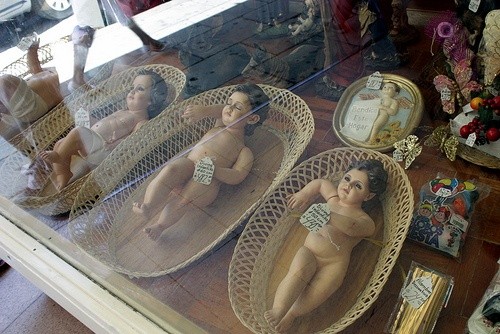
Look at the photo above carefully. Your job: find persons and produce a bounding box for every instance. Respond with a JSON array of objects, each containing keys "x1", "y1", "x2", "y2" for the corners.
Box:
[
  {"x1": 132, "y1": 83, "x2": 270, "y2": 241},
  {"x1": 288, "y1": 6, "x2": 316, "y2": 35},
  {"x1": 266, "y1": 157, "x2": 389, "y2": 331},
  {"x1": 40, "y1": 70, "x2": 167, "y2": 192},
  {"x1": 360, "y1": 80, "x2": 414, "y2": 144}
]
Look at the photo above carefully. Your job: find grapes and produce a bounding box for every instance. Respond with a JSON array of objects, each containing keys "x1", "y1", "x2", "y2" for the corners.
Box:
[{"x1": 475, "y1": 137, "x2": 489, "y2": 146}]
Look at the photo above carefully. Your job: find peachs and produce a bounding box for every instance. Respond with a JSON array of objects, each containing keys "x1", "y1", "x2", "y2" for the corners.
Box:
[{"x1": 470, "y1": 97, "x2": 485, "y2": 110}]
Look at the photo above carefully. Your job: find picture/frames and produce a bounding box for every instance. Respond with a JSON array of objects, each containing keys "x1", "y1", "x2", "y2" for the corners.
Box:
[{"x1": 332, "y1": 74, "x2": 425, "y2": 154}]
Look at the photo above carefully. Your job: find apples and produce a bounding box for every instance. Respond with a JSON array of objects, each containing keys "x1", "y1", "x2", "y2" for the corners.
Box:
[{"x1": 484, "y1": 96, "x2": 500, "y2": 113}]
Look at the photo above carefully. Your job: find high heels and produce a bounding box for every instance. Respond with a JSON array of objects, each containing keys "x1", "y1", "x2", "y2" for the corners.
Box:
[
  {"x1": 146, "y1": 41, "x2": 172, "y2": 57},
  {"x1": 65, "y1": 80, "x2": 96, "y2": 94}
]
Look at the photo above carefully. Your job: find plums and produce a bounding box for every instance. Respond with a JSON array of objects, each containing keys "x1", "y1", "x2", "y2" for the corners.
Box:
[{"x1": 460, "y1": 117, "x2": 499, "y2": 141}]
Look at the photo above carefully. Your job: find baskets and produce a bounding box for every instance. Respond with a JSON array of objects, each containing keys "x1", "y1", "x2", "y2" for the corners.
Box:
[
  {"x1": 66, "y1": 84, "x2": 315, "y2": 277},
  {"x1": 1, "y1": 25, "x2": 117, "y2": 148},
  {"x1": 229, "y1": 147, "x2": 415, "y2": 334},
  {"x1": 0, "y1": 64, "x2": 187, "y2": 218}
]
